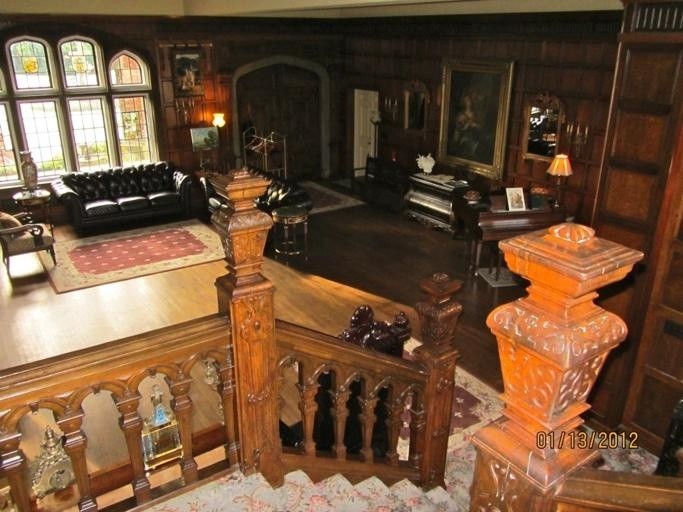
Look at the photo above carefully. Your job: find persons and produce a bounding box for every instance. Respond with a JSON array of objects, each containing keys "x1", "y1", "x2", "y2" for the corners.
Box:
[
  {"x1": 453, "y1": 94, "x2": 490, "y2": 164},
  {"x1": 510, "y1": 192, "x2": 523, "y2": 208}
]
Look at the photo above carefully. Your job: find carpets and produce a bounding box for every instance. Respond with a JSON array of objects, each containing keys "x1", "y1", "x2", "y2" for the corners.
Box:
[
  {"x1": 478, "y1": 267, "x2": 519, "y2": 287},
  {"x1": 365, "y1": 320, "x2": 505, "y2": 462},
  {"x1": 37, "y1": 217, "x2": 226, "y2": 294},
  {"x1": 286, "y1": 180, "x2": 368, "y2": 216}
]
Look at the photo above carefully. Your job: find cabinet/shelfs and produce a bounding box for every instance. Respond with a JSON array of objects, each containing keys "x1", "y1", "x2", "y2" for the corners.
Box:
[{"x1": 244, "y1": 126, "x2": 287, "y2": 179}]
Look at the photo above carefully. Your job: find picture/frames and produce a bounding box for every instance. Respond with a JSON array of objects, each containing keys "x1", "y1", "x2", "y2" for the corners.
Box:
[
  {"x1": 170, "y1": 46, "x2": 204, "y2": 97},
  {"x1": 505, "y1": 187, "x2": 526, "y2": 211},
  {"x1": 435, "y1": 58, "x2": 516, "y2": 182}
]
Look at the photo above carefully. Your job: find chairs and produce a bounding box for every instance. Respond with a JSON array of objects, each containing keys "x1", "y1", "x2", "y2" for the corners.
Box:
[{"x1": 0, "y1": 211, "x2": 56, "y2": 281}]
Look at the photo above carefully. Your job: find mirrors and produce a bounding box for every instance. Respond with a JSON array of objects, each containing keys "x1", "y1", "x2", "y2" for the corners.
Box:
[
  {"x1": 521, "y1": 93, "x2": 562, "y2": 163},
  {"x1": 402, "y1": 76, "x2": 431, "y2": 143}
]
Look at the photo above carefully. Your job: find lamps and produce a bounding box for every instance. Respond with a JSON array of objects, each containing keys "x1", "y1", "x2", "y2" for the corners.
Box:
[{"x1": 546, "y1": 153, "x2": 574, "y2": 209}]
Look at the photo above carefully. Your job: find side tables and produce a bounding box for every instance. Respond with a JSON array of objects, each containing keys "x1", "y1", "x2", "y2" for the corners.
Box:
[
  {"x1": 271, "y1": 204, "x2": 312, "y2": 265},
  {"x1": 12, "y1": 188, "x2": 51, "y2": 213}
]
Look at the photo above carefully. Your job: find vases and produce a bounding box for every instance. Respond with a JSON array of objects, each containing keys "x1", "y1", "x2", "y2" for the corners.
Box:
[{"x1": 20, "y1": 151, "x2": 37, "y2": 190}]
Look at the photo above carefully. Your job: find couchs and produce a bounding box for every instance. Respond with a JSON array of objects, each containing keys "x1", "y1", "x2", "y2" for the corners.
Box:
[
  {"x1": 49, "y1": 161, "x2": 204, "y2": 237},
  {"x1": 206, "y1": 165, "x2": 313, "y2": 224}
]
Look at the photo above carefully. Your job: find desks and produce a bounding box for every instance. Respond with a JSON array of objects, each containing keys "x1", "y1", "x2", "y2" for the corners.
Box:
[{"x1": 403, "y1": 172, "x2": 482, "y2": 240}]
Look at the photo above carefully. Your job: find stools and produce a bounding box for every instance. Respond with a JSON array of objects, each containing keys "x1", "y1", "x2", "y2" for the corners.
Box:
[{"x1": 489, "y1": 247, "x2": 505, "y2": 282}]
